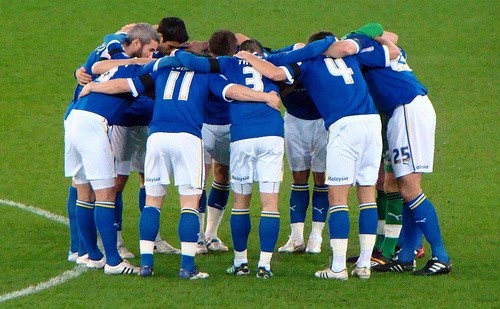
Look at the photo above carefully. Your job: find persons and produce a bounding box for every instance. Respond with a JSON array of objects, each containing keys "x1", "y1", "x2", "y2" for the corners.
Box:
[{"x1": 63, "y1": 16, "x2": 453, "y2": 280}]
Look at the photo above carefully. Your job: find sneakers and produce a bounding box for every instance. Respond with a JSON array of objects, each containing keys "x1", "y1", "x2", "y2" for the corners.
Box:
[
  {"x1": 205, "y1": 237, "x2": 229, "y2": 251},
  {"x1": 372, "y1": 260, "x2": 416, "y2": 272},
  {"x1": 196, "y1": 240, "x2": 208, "y2": 253},
  {"x1": 86, "y1": 256, "x2": 105, "y2": 269},
  {"x1": 348, "y1": 250, "x2": 382, "y2": 263},
  {"x1": 256, "y1": 267, "x2": 273, "y2": 278},
  {"x1": 154, "y1": 240, "x2": 181, "y2": 255},
  {"x1": 351, "y1": 268, "x2": 371, "y2": 279},
  {"x1": 352, "y1": 254, "x2": 392, "y2": 268},
  {"x1": 118, "y1": 246, "x2": 135, "y2": 259},
  {"x1": 69, "y1": 251, "x2": 78, "y2": 262},
  {"x1": 139, "y1": 266, "x2": 154, "y2": 276},
  {"x1": 416, "y1": 247, "x2": 425, "y2": 259},
  {"x1": 179, "y1": 268, "x2": 210, "y2": 279},
  {"x1": 414, "y1": 256, "x2": 453, "y2": 276},
  {"x1": 306, "y1": 235, "x2": 322, "y2": 253},
  {"x1": 227, "y1": 263, "x2": 250, "y2": 275},
  {"x1": 277, "y1": 236, "x2": 306, "y2": 253},
  {"x1": 76, "y1": 254, "x2": 88, "y2": 265},
  {"x1": 315, "y1": 267, "x2": 348, "y2": 280},
  {"x1": 104, "y1": 260, "x2": 140, "y2": 274}
]
